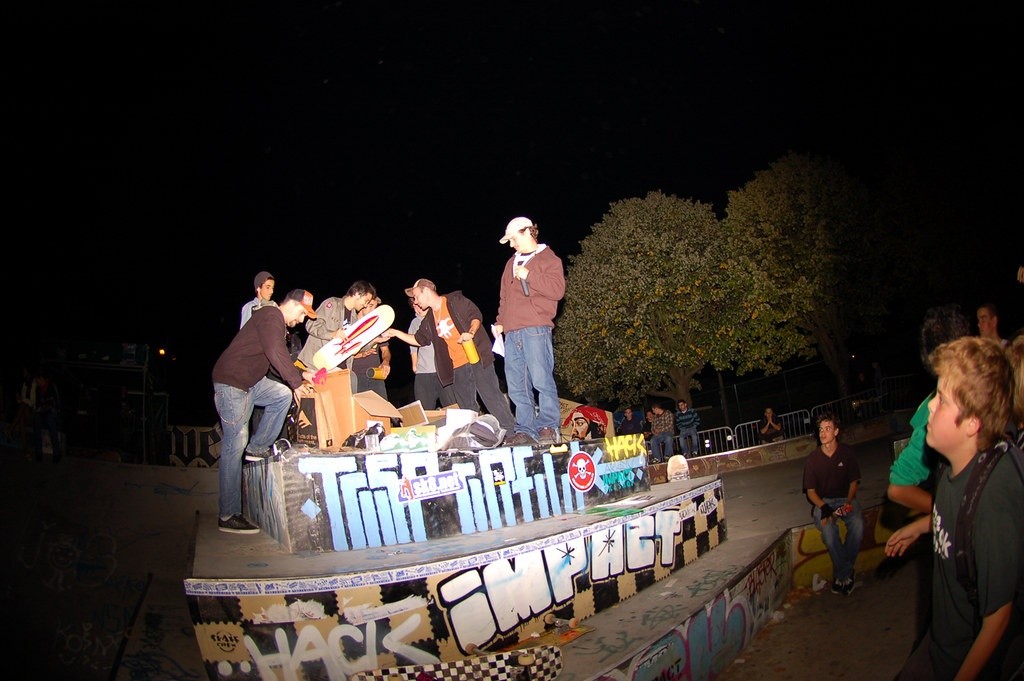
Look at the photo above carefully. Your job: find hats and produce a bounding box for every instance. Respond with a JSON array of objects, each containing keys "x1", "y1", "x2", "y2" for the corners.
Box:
[
  {"x1": 405, "y1": 279, "x2": 436, "y2": 298},
  {"x1": 254, "y1": 271, "x2": 274, "y2": 290},
  {"x1": 287, "y1": 288, "x2": 318, "y2": 319},
  {"x1": 499, "y1": 217, "x2": 532, "y2": 244}
]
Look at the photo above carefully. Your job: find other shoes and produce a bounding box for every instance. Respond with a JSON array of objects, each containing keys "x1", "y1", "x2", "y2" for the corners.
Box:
[
  {"x1": 684, "y1": 454, "x2": 688, "y2": 459},
  {"x1": 665, "y1": 457, "x2": 669, "y2": 463},
  {"x1": 653, "y1": 458, "x2": 658, "y2": 463},
  {"x1": 692, "y1": 453, "x2": 697, "y2": 457}
]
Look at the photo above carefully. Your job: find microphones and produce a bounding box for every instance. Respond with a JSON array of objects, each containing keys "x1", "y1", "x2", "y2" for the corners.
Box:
[{"x1": 520, "y1": 278, "x2": 530, "y2": 297}]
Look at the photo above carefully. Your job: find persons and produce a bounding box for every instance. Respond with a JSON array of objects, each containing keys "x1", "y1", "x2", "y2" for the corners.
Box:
[
  {"x1": 617, "y1": 407, "x2": 641, "y2": 435},
  {"x1": 803, "y1": 412, "x2": 864, "y2": 596},
  {"x1": 642, "y1": 410, "x2": 654, "y2": 442},
  {"x1": 212, "y1": 289, "x2": 318, "y2": 535},
  {"x1": 674, "y1": 399, "x2": 701, "y2": 459},
  {"x1": 651, "y1": 404, "x2": 675, "y2": 463},
  {"x1": 491, "y1": 217, "x2": 566, "y2": 447},
  {"x1": 240, "y1": 271, "x2": 289, "y2": 452},
  {"x1": 758, "y1": 407, "x2": 785, "y2": 445},
  {"x1": 408, "y1": 298, "x2": 457, "y2": 410},
  {"x1": 380, "y1": 279, "x2": 517, "y2": 439},
  {"x1": 883, "y1": 267, "x2": 1024, "y2": 681},
  {"x1": 1, "y1": 373, "x2": 59, "y2": 464},
  {"x1": 351, "y1": 297, "x2": 391, "y2": 400},
  {"x1": 297, "y1": 279, "x2": 377, "y2": 372}
]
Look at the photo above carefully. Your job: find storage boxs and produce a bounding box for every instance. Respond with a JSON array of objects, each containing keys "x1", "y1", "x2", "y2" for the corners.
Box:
[{"x1": 294, "y1": 369, "x2": 459, "y2": 448}]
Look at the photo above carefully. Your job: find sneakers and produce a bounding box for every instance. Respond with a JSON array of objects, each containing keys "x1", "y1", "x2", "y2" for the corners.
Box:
[
  {"x1": 246, "y1": 451, "x2": 277, "y2": 463},
  {"x1": 830, "y1": 578, "x2": 843, "y2": 594},
  {"x1": 218, "y1": 513, "x2": 260, "y2": 534},
  {"x1": 538, "y1": 427, "x2": 556, "y2": 444},
  {"x1": 844, "y1": 569, "x2": 856, "y2": 596},
  {"x1": 502, "y1": 432, "x2": 539, "y2": 446}
]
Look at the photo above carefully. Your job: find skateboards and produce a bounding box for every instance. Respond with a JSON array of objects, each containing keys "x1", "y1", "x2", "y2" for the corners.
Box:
[
  {"x1": 313, "y1": 305, "x2": 395, "y2": 371},
  {"x1": 666, "y1": 454, "x2": 690, "y2": 482}
]
